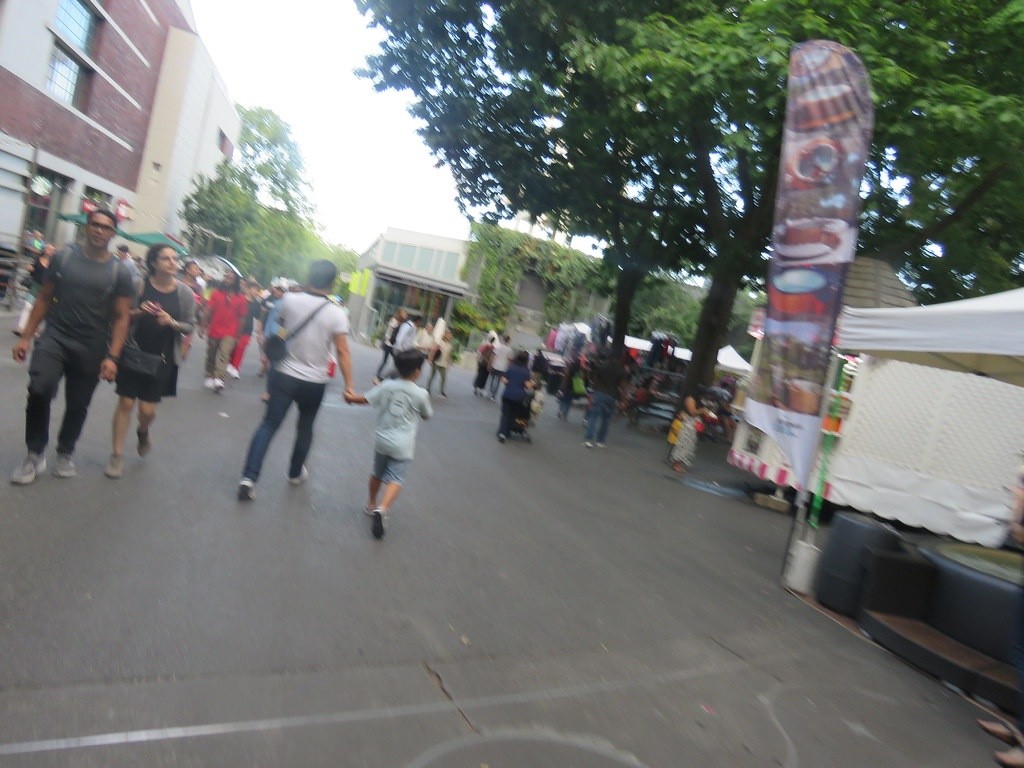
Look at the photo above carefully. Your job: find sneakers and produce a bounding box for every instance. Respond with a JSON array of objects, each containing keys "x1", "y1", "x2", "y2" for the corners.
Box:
[{"x1": 10, "y1": 453, "x2": 46, "y2": 485}]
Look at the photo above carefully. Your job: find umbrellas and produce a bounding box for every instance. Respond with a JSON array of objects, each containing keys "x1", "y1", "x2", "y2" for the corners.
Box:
[{"x1": 56, "y1": 213, "x2": 190, "y2": 256}]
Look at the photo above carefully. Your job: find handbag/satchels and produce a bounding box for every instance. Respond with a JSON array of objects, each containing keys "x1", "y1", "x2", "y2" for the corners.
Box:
[{"x1": 263, "y1": 336, "x2": 288, "y2": 363}]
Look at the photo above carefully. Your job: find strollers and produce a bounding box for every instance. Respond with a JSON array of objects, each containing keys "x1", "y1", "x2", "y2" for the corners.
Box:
[{"x1": 511, "y1": 377, "x2": 543, "y2": 442}]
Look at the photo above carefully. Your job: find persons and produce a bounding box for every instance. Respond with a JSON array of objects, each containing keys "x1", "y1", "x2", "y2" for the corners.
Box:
[
  {"x1": 531, "y1": 349, "x2": 546, "y2": 390},
  {"x1": 496, "y1": 351, "x2": 534, "y2": 441},
  {"x1": 665, "y1": 384, "x2": 710, "y2": 472},
  {"x1": 374, "y1": 309, "x2": 452, "y2": 398},
  {"x1": 11, "y1": 209, "x2": 133, "y2": 484},
  {"x1": 344, "y1": 349, "x2": 433, "y2": 539},
  {"x1": 979, "y1": 474, "x2": 1024, "y2": 768},
  {"x1": 707, "y1": 373, "x2": 738, "y2": 442},
  {"x1": 174, "y1": 262, "x2": 302, "y2": 401},
  {"x1": 473, "y1": 335, "x2": 512, "y2": 402},
  {"x1": 556, "y1": 351, "x2": 670, "y2": 448},
  {"x1": 238, "y1": 260, "x2": 355, "y2": 500},
  {"x1": 106, "y1": 243, "x2": 195, "y2": 479},
  {"x1": 116, "y1": 245, "x2": 148, "y2": 283},
  {"x1": 12, "y1": 225, "x2": 55, "y2": 338}
]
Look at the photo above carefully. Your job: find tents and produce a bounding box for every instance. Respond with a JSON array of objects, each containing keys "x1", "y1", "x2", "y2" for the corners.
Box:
[
  {"x1": 805, "y1": 289, "x2": 1024, "y2": 543},
  {"x1": 716, "y1": 345, "x2": 753, "y2": 378}
]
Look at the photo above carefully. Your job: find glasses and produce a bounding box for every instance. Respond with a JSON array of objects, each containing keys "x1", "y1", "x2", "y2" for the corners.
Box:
[{"x1": 88, "y1": 221, "x2": 117, "y2": 236}]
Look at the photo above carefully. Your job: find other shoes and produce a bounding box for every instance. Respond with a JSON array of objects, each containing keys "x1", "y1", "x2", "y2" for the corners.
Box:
[
  {"x1": 237, "y1": 480, "x2": 255, "y2": 501},
  {"x1": 214, "y1": 378, "x2": 224, "y2": 390},
  {"x1": 137, "y1": 428, "x2": 150, "y2": 456},
  {"x1": 364, "y1": 505, "x2": 377, "y2": 517},
  {"x1": 286, "y1": 466, "x2": 308, "y2": 484},
  {"x1": 105, "y1": 454, "x2": 122, "y2": 477},
  {"x1": 204, "y1": 379, "x2": 214, "y2": 390},
  {"x1": 371, "y1": 510, "x2": 387, "y2": 540},
  {"x1": 226, "y1": 366, "x2": 240, "y2": 378}
]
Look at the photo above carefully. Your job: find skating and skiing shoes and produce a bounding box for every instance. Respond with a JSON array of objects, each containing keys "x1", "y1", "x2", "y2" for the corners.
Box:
[{"x1": 55, "y1": 453, "x2": 76, "y2": 477}]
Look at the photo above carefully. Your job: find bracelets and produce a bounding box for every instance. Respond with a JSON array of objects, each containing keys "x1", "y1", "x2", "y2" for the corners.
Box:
[{"x1": 105, "y1": 354, "x2": 120, "y2": 364}]
